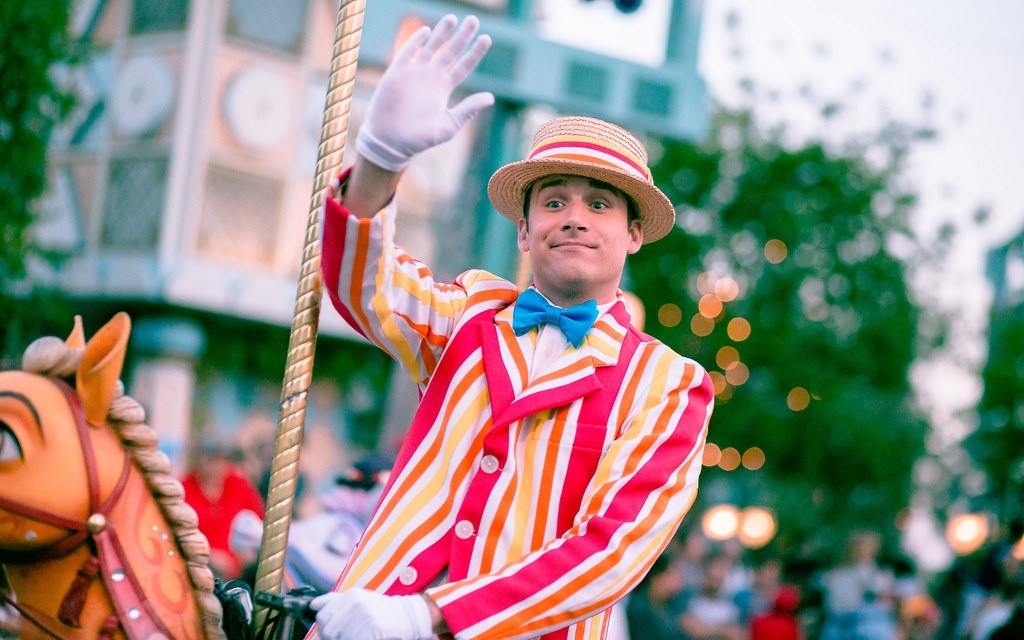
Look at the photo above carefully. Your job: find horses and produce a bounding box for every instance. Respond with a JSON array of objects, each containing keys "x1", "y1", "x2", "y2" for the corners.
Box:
[{"x1": 0, "y1": 312, "x2": 226, "y2": 640}]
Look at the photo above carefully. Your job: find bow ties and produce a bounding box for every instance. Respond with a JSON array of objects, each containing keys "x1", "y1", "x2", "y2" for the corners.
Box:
[{"x1": 512, "y1": 287, "x2": 604, "y2": 347}]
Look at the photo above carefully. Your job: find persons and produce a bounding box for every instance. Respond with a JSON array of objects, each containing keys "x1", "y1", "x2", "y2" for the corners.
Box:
[
  {"x1": 629, "y1": 515, "x2": 1024, "y2": 640},
  {"x1": 304, "y1": 12, "x2": 716, "y2": 640},
  {"x1": 181, "y1": 416, "x2": 307, "y2": 582}
]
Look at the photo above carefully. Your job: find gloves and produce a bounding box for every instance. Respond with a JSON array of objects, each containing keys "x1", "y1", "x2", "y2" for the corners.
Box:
[
  {"x1": 355, "y1": 12, "x2": 499, "y2": 174},
  {"x1": 309, "y1": 587, "x2": 435, "y2": 640}
]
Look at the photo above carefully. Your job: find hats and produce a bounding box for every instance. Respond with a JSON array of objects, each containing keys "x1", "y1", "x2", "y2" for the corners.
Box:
[{"x1": 485, "y1": 114, "x2": 677, "y2": 244}]
